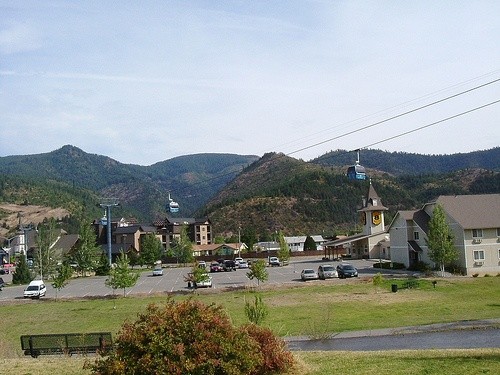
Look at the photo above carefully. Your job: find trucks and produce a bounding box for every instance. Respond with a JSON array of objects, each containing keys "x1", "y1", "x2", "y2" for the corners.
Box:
[{"x1": 269, "y1": 257, "x2": 280, "y2": 267}]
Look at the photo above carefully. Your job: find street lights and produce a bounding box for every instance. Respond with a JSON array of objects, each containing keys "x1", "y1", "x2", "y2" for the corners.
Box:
[{"x1": 0, "y1": 236, "x2": 21, "y2": 264}]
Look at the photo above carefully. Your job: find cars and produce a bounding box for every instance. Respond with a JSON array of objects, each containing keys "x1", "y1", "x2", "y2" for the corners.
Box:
[
  {"x1": 152, "y1": 267, "x2": 163, "y2": 276},
  {"x1": 198, "y1": 261, "x2": 207, "y2": 268},
  {"x1": 301, "y1": 268, "x2": 318, "y2": 281},
  {"x1": 70, "y1": 262, "x2": 79, "y2": 270},
  {"x1": 193, "y1": 273, "x2": 213, "y2": 288},
  {"x1": 223, "y1": 257, "x2": 249, "y2": 272},
  {"x1": 27, "y1": 260, "x2": 34, "y2": 268}
]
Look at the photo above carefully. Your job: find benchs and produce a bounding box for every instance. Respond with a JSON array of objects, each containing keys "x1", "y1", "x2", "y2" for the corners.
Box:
[{"x1": 20, "y1": 331, "x2": 118, "y2": 359}]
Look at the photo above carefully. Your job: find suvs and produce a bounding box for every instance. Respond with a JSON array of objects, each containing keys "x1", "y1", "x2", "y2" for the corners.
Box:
[
  {"x1": 23, "y1": 280, "x2": 47, "y2": 299},
  {"x1": 318, "y1": 263, "x2": 339, "y2": 280},
  {"x1": 336, "y1": 262, "x2": 358, "y2": 279},
  {"x1": 209, "y1": 262, "x2": 222, "y2": 273}
]
